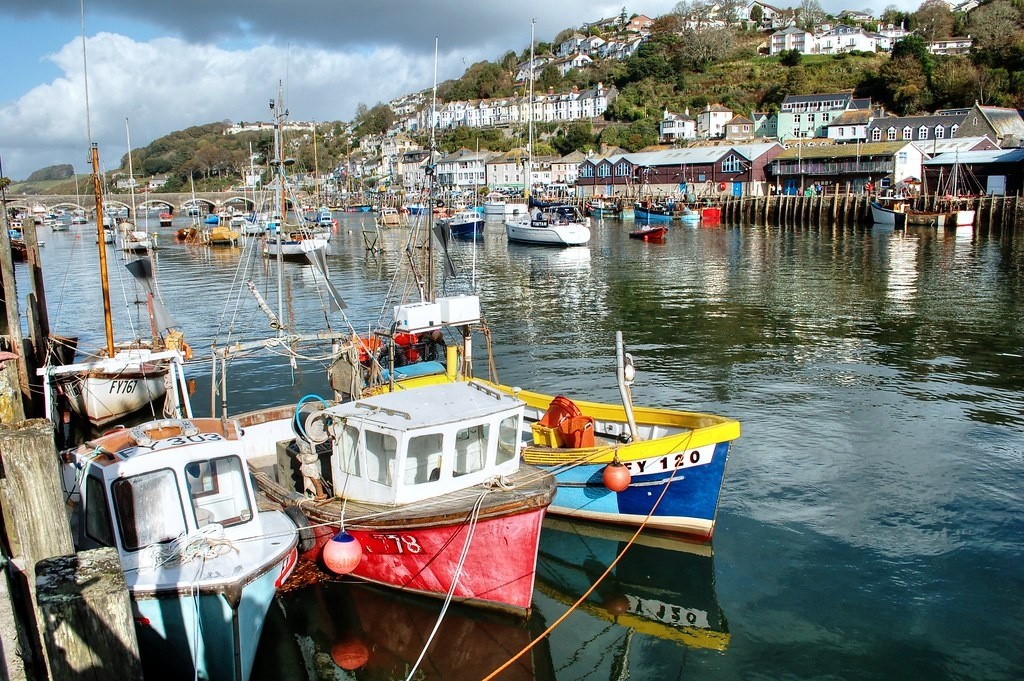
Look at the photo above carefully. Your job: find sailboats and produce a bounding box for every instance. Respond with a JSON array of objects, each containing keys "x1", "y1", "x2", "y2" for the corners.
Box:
[
  {"x1": 114, "y1": 113, "x2": 159, "y2": 251},
  {"x1": 56, "y1": 1, "x2": 185, "y2": 428},
  {"x1": 260, "y1": 79, "x2": 330, "y2": 259}
]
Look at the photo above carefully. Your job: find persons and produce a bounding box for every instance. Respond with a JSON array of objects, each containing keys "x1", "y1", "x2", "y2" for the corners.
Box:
[
  {"x1": 810, "y1": 182, "x2": 823, "y2": 196},
  {"x1": 0, "y1": 351, "x2": 20, "y2": 370},
  {"x1": 863, "y1": 182, "x2": 873, "y2": 196}
]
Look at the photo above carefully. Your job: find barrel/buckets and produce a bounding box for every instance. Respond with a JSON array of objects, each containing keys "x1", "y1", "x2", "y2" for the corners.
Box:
[
  {"x1": 537, "y1": 394, "x2": 582, "y2": 427},
  {"x1": 558, "y1": 416, "x2": 595, "y2": 448}
]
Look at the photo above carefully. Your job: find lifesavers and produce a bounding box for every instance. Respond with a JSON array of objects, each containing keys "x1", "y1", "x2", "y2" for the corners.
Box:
[
  {"x1": 131, "y1": 417, "x2": 198, "y2": 448},
  {"x1": 284, "y1": 505, "x2": 317, "y2": 551}
]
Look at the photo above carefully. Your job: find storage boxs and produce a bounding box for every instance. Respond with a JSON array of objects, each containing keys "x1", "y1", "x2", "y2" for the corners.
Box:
[
  {"x1": 530, "y1": 422, "x2": 564, "y2": 449},
  {"x1": 436, "y1": 296, "x2": 480, "y2": 326},
  {"x1": 394, "y1": 301, "x2": 441, "y2": 335}
]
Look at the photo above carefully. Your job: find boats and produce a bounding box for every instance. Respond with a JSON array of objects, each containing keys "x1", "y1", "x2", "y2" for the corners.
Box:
[
  {"x1": 290, "y1": 574, "x2": 557, "y2": 681},
  {"x1": 503, "y1": 202, "x2": 590, "y2": 246},
  {"x1": 318, "y1": 37, "x2": 743, "y2": 537},
  {"x1": 435, "y1": 207, "x2": 486, "y2": 241},
  {"x1": 159, "y1": 211, "x2": 174, "y2": 227},
  {"x1": 16, "y1": 19, "x2": 722, "y2": 235},
  {"x1": 210, "y1": 141, "x2": 559, "y2": 617},
  {"x1": 869, "y1": 148, "x2": 985, "y2": 227},
  {"x1": 374, "y1": 207, "x2": 399, "y2": 225},
  {"x1": 51, "y1": 343, "x2": 299, "y2": 681},
  {"x1": 533, "y1": 518, "x2": 733, "y2": 681},
  {"x1": 629, "y1": 225, "x2": 668, "y2": 239}
]
